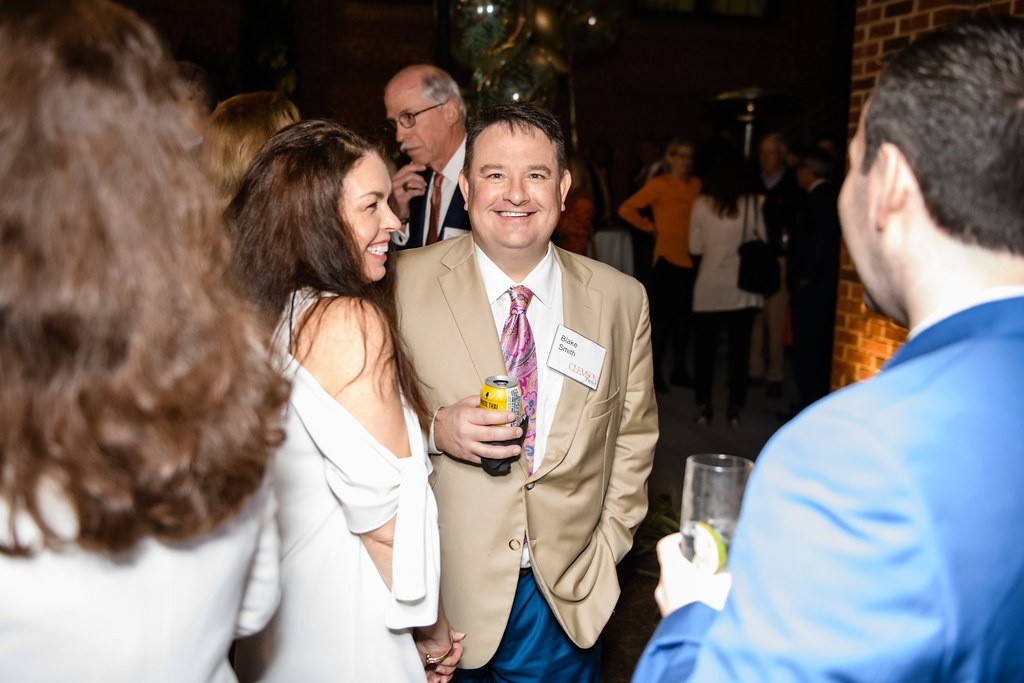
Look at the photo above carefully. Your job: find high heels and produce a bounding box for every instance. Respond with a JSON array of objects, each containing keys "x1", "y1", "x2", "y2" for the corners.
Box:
[
  {"x1": 725, "y1": 406, "x2": 741, "y2": 424},
  {"x1": 693, "y1": 405, "x2": 715, "y2": 426}
]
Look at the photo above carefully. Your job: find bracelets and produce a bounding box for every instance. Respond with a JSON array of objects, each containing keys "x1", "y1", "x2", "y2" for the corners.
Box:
[
  {"x1": 398, "y1": 214, "x2": 411, "y2": 226},
  {"x1": 425, "y1": 627, "x2": 455, "y2": 666}
]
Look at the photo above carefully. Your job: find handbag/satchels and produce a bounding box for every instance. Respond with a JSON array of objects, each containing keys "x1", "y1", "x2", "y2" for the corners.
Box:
[{"x1": 737, "y1": 193, "x2": 782, "y2": 299}]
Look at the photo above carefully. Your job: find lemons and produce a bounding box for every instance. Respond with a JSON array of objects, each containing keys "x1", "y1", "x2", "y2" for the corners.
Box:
[{"x1": 694, "y1": 521, "x2": 725, "y2": 574}]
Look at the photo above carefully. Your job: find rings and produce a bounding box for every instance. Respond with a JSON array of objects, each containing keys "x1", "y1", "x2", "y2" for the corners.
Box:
[{"x1": 403, "y1": 180, "x2": 409, "y2": 192}]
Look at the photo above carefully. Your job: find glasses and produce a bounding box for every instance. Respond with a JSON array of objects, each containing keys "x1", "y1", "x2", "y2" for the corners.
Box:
[{"x1": 384, "y1": 103, "x2": 444, "y2": 133}]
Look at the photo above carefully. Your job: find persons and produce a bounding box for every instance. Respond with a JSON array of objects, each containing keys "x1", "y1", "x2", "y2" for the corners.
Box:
[
  {"x1": 210, "y1": 114, "x2": 466, "y2": 683},
  {"x1": 544, "y1": 128, "x2": 853, "y2": 436},
  {"x1": 629, "y1": 14, "x2": 1024, "y2": 683},
  {"x1": 0, "y1": 2, "x2": 284, "y2": 682},
  {"x1": 198, "y1": 91, "x2": 305, "y2": 243},
  {"x1": 365, "y1": 64, "x2": 490, "y2": 266},
  {"x1": 378, "y1": 99, "x2": 658, "y2": 682}
]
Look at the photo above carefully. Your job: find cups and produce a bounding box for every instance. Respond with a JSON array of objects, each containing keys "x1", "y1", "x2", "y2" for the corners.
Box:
[{"x1": 680, "y1": 454, "x2": 754, "y2": 572}]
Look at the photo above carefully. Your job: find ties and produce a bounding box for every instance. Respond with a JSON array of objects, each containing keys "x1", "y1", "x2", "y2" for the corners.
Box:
[
  {"x1": 425, "y1": 172, "x2": 445, "y2": 246},
  {"x1": 499, "y1": 284, "x2": 538, "y2": 476}
]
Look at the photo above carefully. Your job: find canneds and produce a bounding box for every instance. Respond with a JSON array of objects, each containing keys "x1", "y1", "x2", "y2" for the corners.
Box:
[{"x1": 479, "y1": 375, "x2": 521, "y2": 429}]
